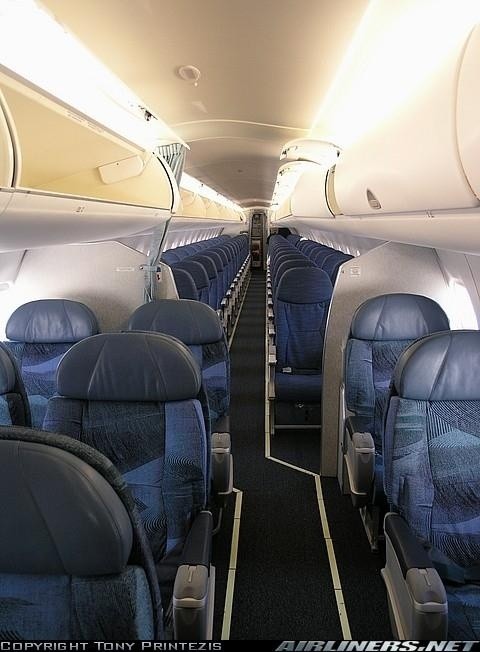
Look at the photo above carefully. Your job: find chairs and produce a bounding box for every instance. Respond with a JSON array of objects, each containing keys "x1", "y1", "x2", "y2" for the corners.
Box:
[{"x1": 0, "y1": 234, "x2": 480, "y2": 652}]
